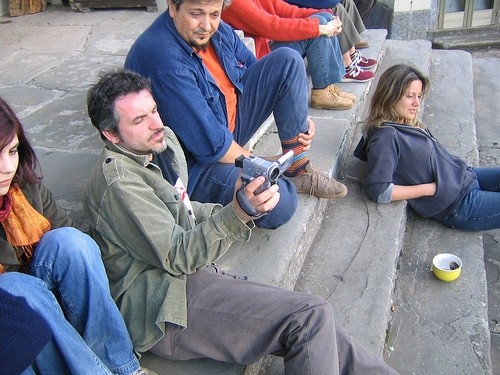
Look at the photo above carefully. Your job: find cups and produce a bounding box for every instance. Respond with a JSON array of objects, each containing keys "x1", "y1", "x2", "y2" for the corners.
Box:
[{"x1": 430, "y1": 253, "x2": 462, "y2": 282}]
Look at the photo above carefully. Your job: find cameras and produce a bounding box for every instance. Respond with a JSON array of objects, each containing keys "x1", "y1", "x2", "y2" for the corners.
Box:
[{"x1": 235, "y1": 149, "x2": 294, "y2": 196}]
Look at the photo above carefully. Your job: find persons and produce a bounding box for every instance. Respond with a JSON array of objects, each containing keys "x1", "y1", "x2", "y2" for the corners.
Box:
[
  {"x1": 221, "y1": 0, "x2": 357, "y2": 111},
  {"x1": 83, "y1": 69, "x2": 401, "y2": 374},
  {"x1": 353, "y1": 65, "x2": 500, "y2": 232},
  {"x1": 0, "y1": 96, "x2": 157, "y2": 375},
  {"x1": 288, "y1": 0, "x2": 377, "y2": 81},
  {"x1": 125, "y1": 0, "x2": 347, "y2": 230}
]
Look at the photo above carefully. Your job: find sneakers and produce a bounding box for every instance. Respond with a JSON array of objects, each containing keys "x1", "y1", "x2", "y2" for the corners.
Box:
[
  {"x1": 355, "y1": 39, "x2": 370, "y2": 49},
  {"x1": 294, "y1": 163, "x2": 348, "y2": 199},
  {"x1": 310, "y1": 85, "x2": 354, "y2": 110},
  {"x1": 331, "y1": 83, "x2": 357, "y2": 102},
  {"x1": 355, "y1": 53, "x2": 378, "y2": 69},
  {"x1": 342, "y1": 63, "x2": 374, "y2": 83}
]
